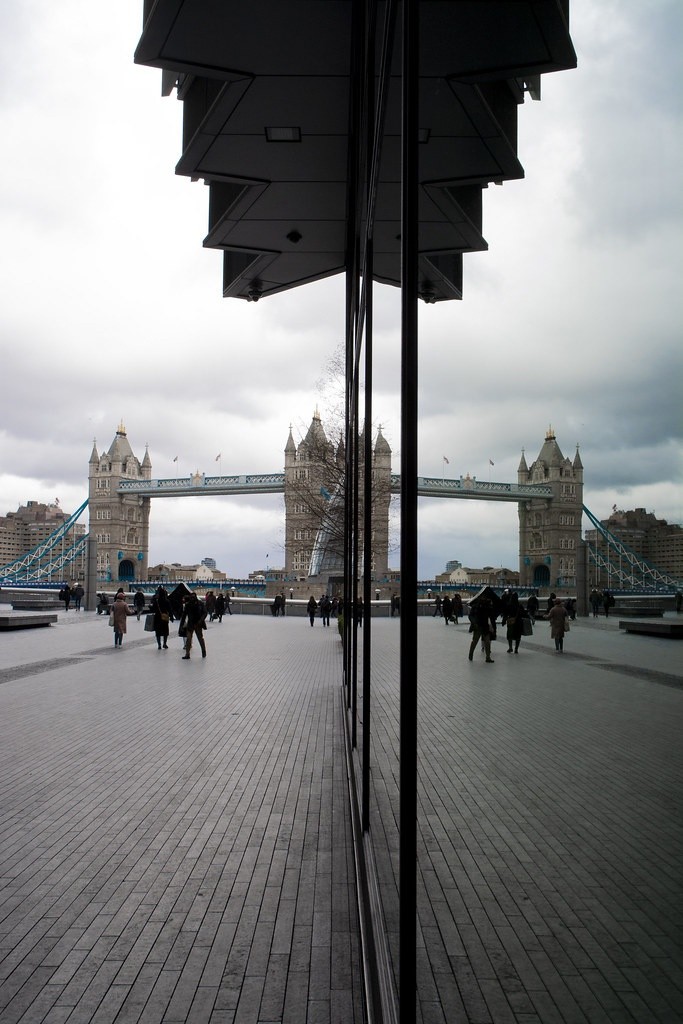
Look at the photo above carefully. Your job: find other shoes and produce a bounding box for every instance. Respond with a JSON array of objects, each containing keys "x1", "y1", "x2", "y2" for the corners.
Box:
[
  {"x1": 202, "y1": 647, "x2": 206, "y2": 657},
  {"x1": 115, "y1": 644, "x2": 117, "y2": 648},
  {"x1": 507, "y1": 648, "x2": 513, "y2": 652},
  {"x1": 469, "y1": 651, "x2": 473, "y2": 661},
  {"x1": 158, "y1": 646, "x2": 161, "y2": 649},
  {"x1": 163, "y1": 645, "x2": 168, "y2": 649},
  {"x1": 515, "y1": 649, "x2": 518, "y2": 653},
  {"x1": 182, "y1": 655, "x2": 190, "y2": 659},
  {"x1": 119, "y1": 646, "x2": 122, "y2": 649},
  {"x1": 485, "y1": 658, "x2": 495, "y2": 662}
]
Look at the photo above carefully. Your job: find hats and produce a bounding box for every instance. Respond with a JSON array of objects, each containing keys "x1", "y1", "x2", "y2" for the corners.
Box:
[{"x1": 117, "y1": 592, "x2": 126, "y2": 600}]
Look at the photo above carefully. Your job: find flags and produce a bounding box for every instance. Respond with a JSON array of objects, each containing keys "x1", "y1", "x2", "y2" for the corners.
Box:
[
  {"x1": 173, "y1": 456, "x2": 178, "y2": 463},
  {"x1": 490, "y1": 460, "x2": 494, "y2": 466},
  {"x1": 54, "y1": 498, "x2": 60, "y2": 506},
  {"x1": 215, "y1": 453, "x2": 221, "y2": 461},
  {"x1": 444, "y1": 455, "x2": 450, "y2": 464}
]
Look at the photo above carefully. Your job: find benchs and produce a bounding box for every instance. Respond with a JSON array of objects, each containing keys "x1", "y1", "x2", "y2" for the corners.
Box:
[
  {"x1": 103, "y1": 603, "x2": 153, "y2": 615},
  {"x1": 524, "y1": 609, "x2": 547, "y2": 620}
]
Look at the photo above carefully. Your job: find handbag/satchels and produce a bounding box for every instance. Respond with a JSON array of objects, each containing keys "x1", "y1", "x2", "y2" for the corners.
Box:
[
  {"x1": 109, "y1": 611, "x2": 115, "y2": 626},
  {"x1": 144, "y1": 615, "x2": 156, "y2": 631},
  {"x1": 564, "y1": 619, "x2": 570, "y2": 632},
  {"x1": 522, "y1": 612, "x2": 533, "y2": 635},
  {"x1": 162, "y1": 614, "x2": 169, "y2": 621}
]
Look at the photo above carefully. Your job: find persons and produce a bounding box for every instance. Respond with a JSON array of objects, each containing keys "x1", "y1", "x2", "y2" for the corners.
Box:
[
  {"x1": 96, "y1": 587, "x2": 135, "y2": 649},
  {"x1": 307, "y1": 595, "x2": 343, "y2": 627},
  {"x1": 589, "y1": 588, "x2": 612, "y2": 618},
  {"x1": 272, "y1": 591, "x2": 285, "y2": 617},
  {"x1": 432, "y1": 594, "x2": 464, "y2": 625},
  {"x1": 357, "y1": 597, "x2": 363, "y2": 627},
  {"x1": 180, "y1": 595, "x2": 192, "y2": 649},
  {"x1": 675, "y1": 589, "x2": 683, "y2": 614},
  {"x1": 205, "y1": 591, "x2": 217, "y2": 622},
  {"x1": 62, "y1": 584, "x2": 84, "y2": 611},
  {"x1": 180, "y1": 592, "x2": 208, "y2": 659},
  {"x1": 173, "y1": 590, "x2": 182, "y2": 620},
  {"x1": 133, "y1": 587, "x2": 146, "y2": 621},
  {"x1": 390, "y1": 592, "x2": 400, "y2": 617},
  {"x1": 215, "y1": 593, "x2": 226, "y2": 623},
  {"x1": 148, "y1": 589, "x2": 174, "y2": 650},
  {"x1": 501, "y1": 588, "x2": 576, "y2": 654},
  {"x1": 468, "y1": 594, "x2": 497, "y2": 663},
  {"x1": 224, "y1": 593, "x2": 233, "y2": 615},
  {"x1": 150, "y1": 585, "x2": 168, "y2": 605}
]
